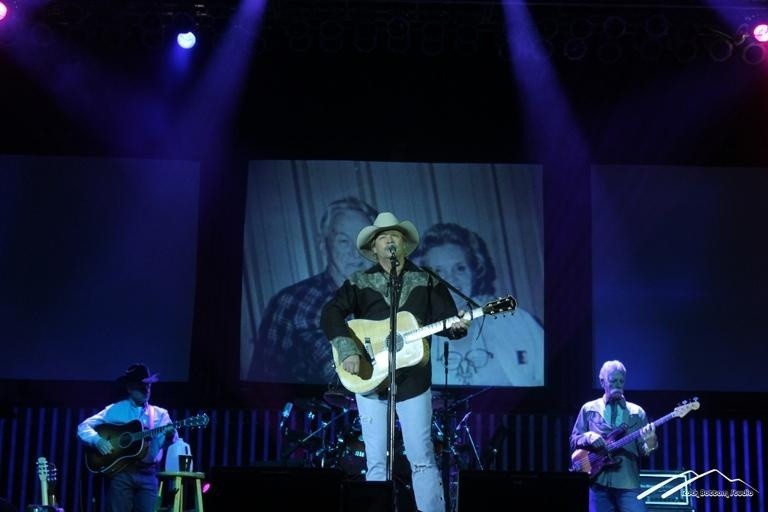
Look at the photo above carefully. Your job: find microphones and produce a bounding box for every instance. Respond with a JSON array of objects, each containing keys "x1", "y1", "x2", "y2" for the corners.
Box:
[{"x1": 385, "y1": 243, "x2": 398, "y2": 258}]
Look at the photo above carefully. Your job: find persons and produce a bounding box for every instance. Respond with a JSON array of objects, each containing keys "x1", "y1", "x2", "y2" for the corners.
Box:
[
  {"x1": 319, "y1": 211, "x2": 473, "y2": 512},
  {"x1": 567, "y1": 359, "x2": 660, "y2": 512},
  {"x1": 77, "y1": 362, "x2": 179, "y2": 511},
  {"x1": 418, "y1": 222, "x2": 545, "y2": 386},
  {"x1": 247, "y1": 194, "x2": 381, "y2": 383}
]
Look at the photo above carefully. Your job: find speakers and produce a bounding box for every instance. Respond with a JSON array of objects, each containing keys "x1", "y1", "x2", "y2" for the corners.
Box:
[
  {"x1": 460, "y1": 471, "x2": 589, "y2": 512},
  {"x1": 340, "y1": 481, "x2": 392, "y2": 512},
  {"x1": 207, "y1": 466, "x2": 345, "y2": 512}
]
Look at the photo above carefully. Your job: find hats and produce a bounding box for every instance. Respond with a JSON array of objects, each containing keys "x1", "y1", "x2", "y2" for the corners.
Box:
[
  {"x1": 117, "y1": 365, "x2": 159, "y2": 384},
  {"x1": 356, "y1": 212, "x2": 420, "y2": 263}
]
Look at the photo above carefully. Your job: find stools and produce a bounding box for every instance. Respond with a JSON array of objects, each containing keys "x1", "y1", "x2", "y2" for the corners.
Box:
[{"x1": 156, "y1": 472, "x2": 205, "y2": 512}]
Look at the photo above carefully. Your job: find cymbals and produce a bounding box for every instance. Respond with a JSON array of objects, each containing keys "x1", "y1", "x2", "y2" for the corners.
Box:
[{"x1": 326, "y1": 394, "x2": 357, "y2": 409}]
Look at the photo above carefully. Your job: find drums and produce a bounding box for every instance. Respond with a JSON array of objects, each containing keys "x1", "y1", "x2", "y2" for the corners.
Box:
[
  {"x1": 336, "y1": 429, "x2": 367, "y2": 474},
  {"x1": 281, "y1": 403, "x2": 333, "y2": 448}
]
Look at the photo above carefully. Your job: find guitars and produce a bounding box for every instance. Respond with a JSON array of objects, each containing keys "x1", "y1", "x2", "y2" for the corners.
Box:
[
  {"x1": 332, "y1": 295, "x2": 517, "y2": 396},
  {"x1": 85, "y1": 414, "x2": 209, "y2": 476},
  {"x1": 570, "y1": 396, "x2": 700, "y2": 481}
]
